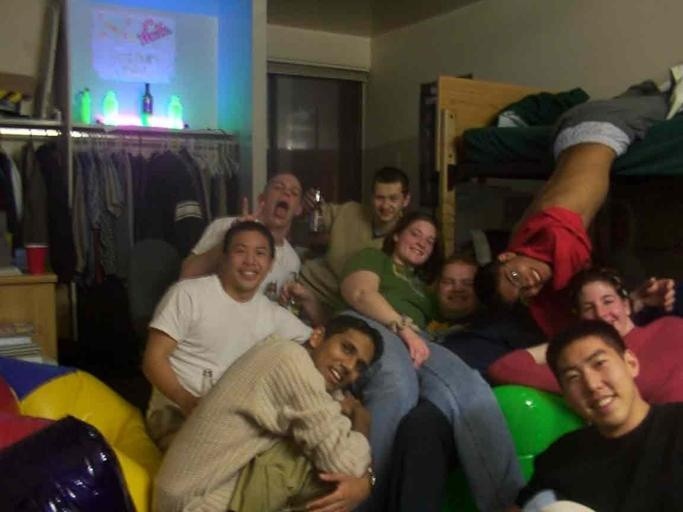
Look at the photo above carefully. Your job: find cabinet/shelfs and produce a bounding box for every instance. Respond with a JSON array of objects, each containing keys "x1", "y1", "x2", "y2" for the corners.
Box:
[{"x1": 0, "y1": 256, "x2": 62, "y2": 364}]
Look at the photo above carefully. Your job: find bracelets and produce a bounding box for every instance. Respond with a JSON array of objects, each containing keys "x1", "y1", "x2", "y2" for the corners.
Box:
[
  {"x1": 364, "y1": 467, "x2": 377, "y2": 488},
  {"x1": 636, "y1": 289, "x2": 650, "y2": 309},
  {"x1": 387, "y1": 312, "x2": 414, "y2": 333}
]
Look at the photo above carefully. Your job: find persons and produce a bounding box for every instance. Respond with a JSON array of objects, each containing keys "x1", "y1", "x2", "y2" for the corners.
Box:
[
  {"x1": 149, "y1": 315, "x2": 384, "y2": 510},
  {"x1": 178, "y1": 174, "x2": 302, "y2": 313},
  {"x1": 519, "y1": 318, "x2": 681, "y2": 511},
  {"x1": 390, "y1": 252, "x2": 544, "y2": 512},
  {"x1": 294, "y1": 166, "x2": 411, "y2": 327},
  {"x1": 486, "y1": 266, "x2": 681, "y2": 403},
  {"x1": 142, "y1": 221, "x2": 326, "y2": 457},
  {"x1": 473, "y1": 66, "x2": 682, "y2": 341},
  {"x1": 333, "y1": 215, "x2": 522, "y2": 512}
]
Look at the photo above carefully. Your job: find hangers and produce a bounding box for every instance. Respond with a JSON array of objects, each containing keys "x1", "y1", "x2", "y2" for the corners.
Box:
[{"x1": 1, "y1": 121, "x2": 243, "y2": 185}]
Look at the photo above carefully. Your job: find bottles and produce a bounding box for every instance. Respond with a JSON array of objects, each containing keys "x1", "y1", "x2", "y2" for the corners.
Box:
[
  {"x1": 306, "y1": 189, "x2": 325, "y2": 234},
  {"x1": 102, "y1": 91, "x2": 117, "y2": 125},
  {"x1": 141, "y1": 83, "x2": 152, "y2": 126},
  {"x1": 200, "y1": 368, "x2": 213, "y2": 395},
  {"x1": 81, "y1": 88, "x2": 91, "y2": 124},
  {"x1": 266, "y1": 278, "x2": 278, "y2": 299},
  {"x1": 168, "y1": 95, "x2": 183, "y2": 129},
  {"x1": 284, "y1": 272, "x2": 302, "y2": 315}
]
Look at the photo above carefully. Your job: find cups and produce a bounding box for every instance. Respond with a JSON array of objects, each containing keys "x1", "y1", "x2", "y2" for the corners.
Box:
[{"x1": 25, "y1": 245, "x2": 46, "y2": 276}]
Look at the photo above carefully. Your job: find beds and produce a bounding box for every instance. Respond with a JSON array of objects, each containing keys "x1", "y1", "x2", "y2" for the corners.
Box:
[{"x1": 429, "y1": 72, "x2": 680, "y2": 264}]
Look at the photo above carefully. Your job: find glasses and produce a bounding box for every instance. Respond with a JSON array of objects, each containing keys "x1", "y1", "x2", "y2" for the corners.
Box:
[{"x1": 502, "y1": 260, "x2": 529, "y2": 311}]
